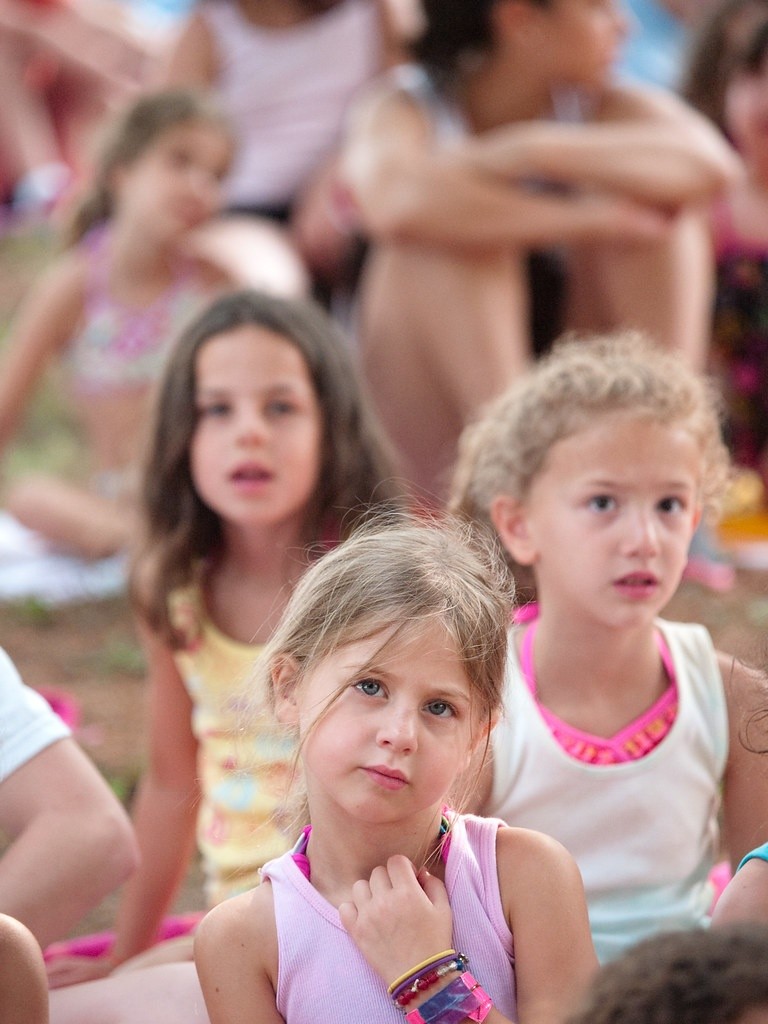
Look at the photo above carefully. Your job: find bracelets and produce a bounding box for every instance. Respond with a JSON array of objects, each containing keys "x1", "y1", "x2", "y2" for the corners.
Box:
[
  {"x1": 736, "y1": 841, "x2": 768, "y2": 867},
  {"x1": 387, "y1": 949, "x2": 495, "y2": 1024}
]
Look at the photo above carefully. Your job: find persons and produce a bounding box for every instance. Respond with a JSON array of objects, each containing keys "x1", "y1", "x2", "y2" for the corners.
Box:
[
  {"x1": 0, "y1": 0, "x2": 768, "y2": 550},
  {"x1": 189, "y1": 494, "x2": 602, "y2": 1024},
  {"x1": 455, "y1": 327, "x2": 768, "y2": 964},
  {"x1": 0, "y1": 645, "x2": 139, "y2": 1023},
  {"x1": 47, "y1": 284, "x2": 412, "y2": 974},
  {"x1": 573, "y1": 934, "x2": 768, "y2": 1024}
]
